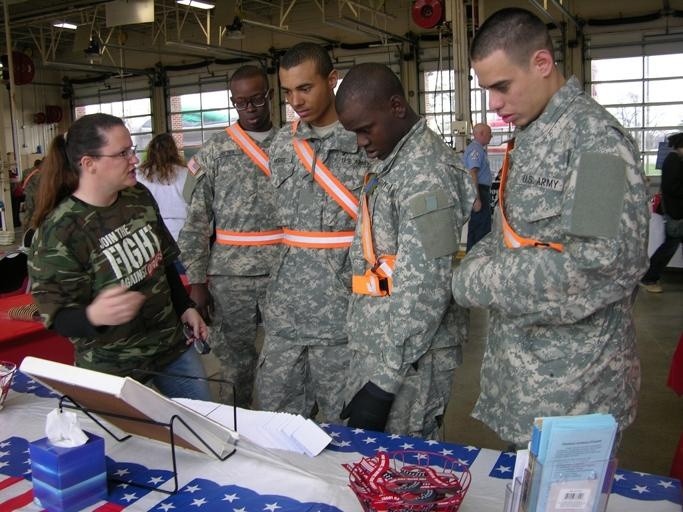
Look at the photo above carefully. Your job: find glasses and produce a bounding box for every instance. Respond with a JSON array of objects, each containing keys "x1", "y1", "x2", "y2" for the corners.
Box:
[
  {"x1": 230, "y1": 87, "x2": 271, "y2": 111},
  {"x1": 182, "y1": 322, "x2": 211, "y2": 355},
  {"x1": 79, "y1": 144, "x2": 137, "y2": 166}
]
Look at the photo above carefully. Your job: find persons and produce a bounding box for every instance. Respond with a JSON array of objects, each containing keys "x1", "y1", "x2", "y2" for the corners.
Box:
[
  {"x1": 22, "y1": 159, "x2": 41, "y2": 233},
  {"x1": 249, "y1": 44, "x2": 373, "y2": 419},
  {"x1": 463, "y1": 123, "x2": 493, "y2": 254},
  {"x1": 336, "y1": 67, "x2": 479, "y2": 441},
  {"x1": 135, "y1": 133, "x2": 188, "y2": 242},
  {"x1": 25, "y1": 113, "x2": 215, "y2": 401},
  {"x1": 451, "y1": 7, "x2": 650, "y2": 453},
  {"x1": 639, "y1": 132, "x2": 683, "y2": 292},
  {"x1": 177, "y1": 67, "x2": 283, "y2": 406}
]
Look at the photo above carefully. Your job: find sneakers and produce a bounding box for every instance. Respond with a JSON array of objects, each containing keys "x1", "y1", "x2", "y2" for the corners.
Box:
[{"x1": 637, "y1": 280, "x2": 664, "y2": 292}]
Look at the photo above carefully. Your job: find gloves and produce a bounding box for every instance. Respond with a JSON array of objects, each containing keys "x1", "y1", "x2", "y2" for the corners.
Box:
[{"x1": 339, "y1": 380, "x2": 395, "y2": 432}]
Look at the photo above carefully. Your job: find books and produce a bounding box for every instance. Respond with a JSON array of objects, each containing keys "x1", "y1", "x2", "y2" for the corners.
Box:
[{"x1": 508, "y1": 412, "x2": 619, "y2": 512}]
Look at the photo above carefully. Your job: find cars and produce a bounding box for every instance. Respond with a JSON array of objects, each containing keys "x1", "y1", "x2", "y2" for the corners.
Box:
[{"x1": 135, "y1": 107, "x2": 237, "y2": 152}]
